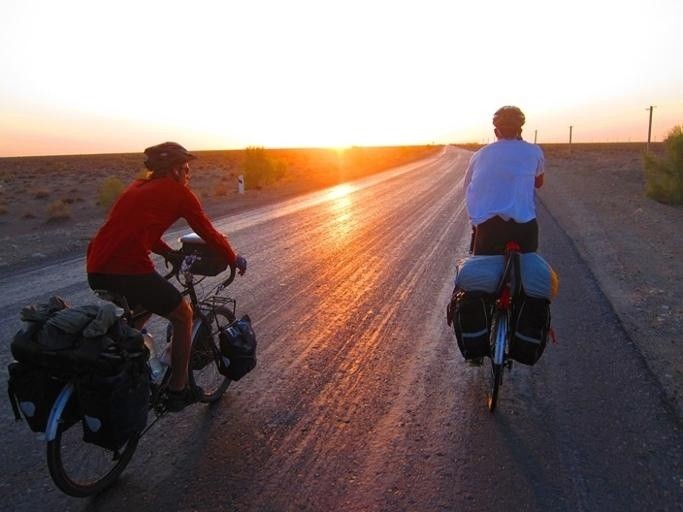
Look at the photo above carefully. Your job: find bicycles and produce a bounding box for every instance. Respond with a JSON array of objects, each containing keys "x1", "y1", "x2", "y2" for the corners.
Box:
[
  {"x1": 43, "y1": 234, "x2": 238, "y2": 496},
  {"x1": 479, "y1": 241, "x2": 526, "y2": 415}
]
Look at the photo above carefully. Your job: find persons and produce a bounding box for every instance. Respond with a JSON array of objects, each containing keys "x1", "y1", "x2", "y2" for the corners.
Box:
[
  {"x1": 459, "y1": 104, "x2": 545, "y2": 367},
  {"x1": 85, "y1": 142, "x2": 247, "y2": 413}
]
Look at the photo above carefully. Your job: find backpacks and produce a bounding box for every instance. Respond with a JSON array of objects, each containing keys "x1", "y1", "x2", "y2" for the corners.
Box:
[
  {"x1": 7, "y1": 360, "x2": 149, "y2": 450},
  {"x1": 209, "y1": 314, "x2": 257, "y2": 381},
  {"x1": 452, "y1": 296, "x2": 551, "y2": 366}
]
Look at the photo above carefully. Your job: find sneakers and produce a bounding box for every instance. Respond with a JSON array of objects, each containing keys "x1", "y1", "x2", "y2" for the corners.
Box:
[{"x1": 165, "y1": 383, "x2": 205, "y2": 412}]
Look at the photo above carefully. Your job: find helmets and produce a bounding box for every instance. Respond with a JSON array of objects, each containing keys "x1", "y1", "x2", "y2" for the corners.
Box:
[
  {"x1": 493, "y1": 106, "x2": 525, "y2": 126},
  {"x1": 143, "y1": 142, "x2": 197, "y2": 171}
]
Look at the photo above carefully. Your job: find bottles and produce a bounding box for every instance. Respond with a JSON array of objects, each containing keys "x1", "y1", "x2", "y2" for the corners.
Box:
[{"x1": 141, "y1": 328, "x2": 165, "y2": 378}]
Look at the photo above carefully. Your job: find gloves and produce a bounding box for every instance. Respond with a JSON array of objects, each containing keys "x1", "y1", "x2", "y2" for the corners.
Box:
[{"x1": 233, "y1": 255, "x2": 247, "y2": 276}]
